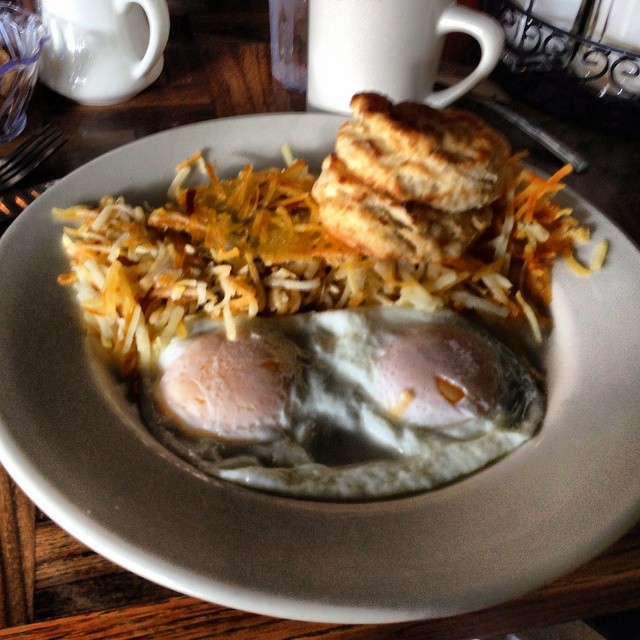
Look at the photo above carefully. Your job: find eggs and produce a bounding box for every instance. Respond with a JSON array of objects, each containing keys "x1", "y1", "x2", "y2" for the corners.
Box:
[
  {"x1": 143, "y1": 316, "x2": 311, "y2": 494},
  {"x1": 311, "y1": 307, "x2": 537, "y2": 499}
]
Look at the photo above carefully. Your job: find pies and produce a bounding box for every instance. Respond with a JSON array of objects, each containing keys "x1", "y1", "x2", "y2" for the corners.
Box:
[{"x1": 316, "y1": 90, "x2": 514, "y2": 263}]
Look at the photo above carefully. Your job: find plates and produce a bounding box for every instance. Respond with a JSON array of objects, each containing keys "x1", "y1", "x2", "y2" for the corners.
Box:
[{"x1": 0, "y1": 109, "x2": 640, "y2": 623}]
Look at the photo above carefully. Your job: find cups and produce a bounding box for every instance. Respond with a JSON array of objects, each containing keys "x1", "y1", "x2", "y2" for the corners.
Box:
[
  {"x1": 35, "y1": 0, "x2": 171, "y2": 107},
  {"x1": 1, "y1": 2, "x2": 51, "y2": 144},
  {"x1": 305, "y1": 1, "x2": 503, "y2": 113}
]
